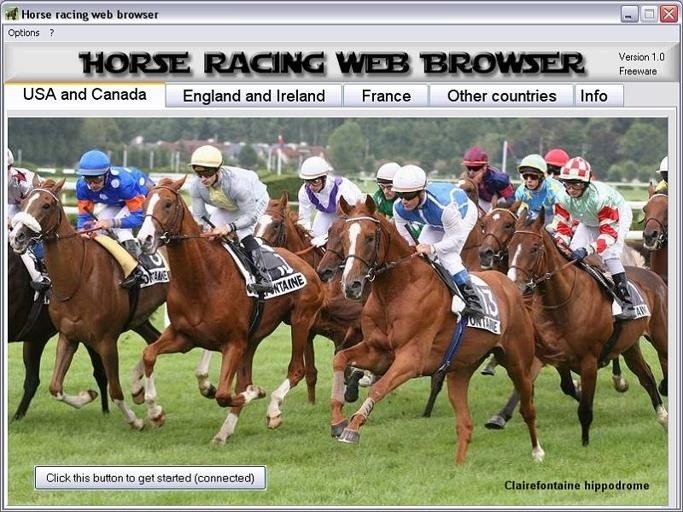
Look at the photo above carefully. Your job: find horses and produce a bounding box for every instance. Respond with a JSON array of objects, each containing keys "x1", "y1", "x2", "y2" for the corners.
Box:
[
  {"x1": 643, "y1": 182, "x2": 669, "y2": 287},
  {"x1": 7, "y1": 242, "x2": 162, "y2": 424},
  {"x1": 480, "y1": 195, "x2": 629, "y2": 402},
  {"x1": 454, "y1": 171, "x2": 507, "y2": 376},
  {"x1": 507, "y1": 207, "x2": 669, "y2": 446},
  {"x1": 253, "y1": 192, "x2": 363, "y2": 405},
  {"x1": 9, "y1": 172, "x2": 217, "y2": 430},
  {"x1": 136, "y1": 174, "x2": 331, "y2": 446},
  {"x1": 331, "y1": 195, "x2": 567, "y2": 465},
  {"x1": 317, "y1": 200, "x2": 447, "y2": 417}
]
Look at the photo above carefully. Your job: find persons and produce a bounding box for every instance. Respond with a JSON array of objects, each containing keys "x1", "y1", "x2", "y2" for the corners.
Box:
[
  {"x1": 543, "y1": 148, "x2": 569, "y2": 181},
  {"x1": 460, "y1": 147, "x2": 515, "y2": 209},
  {"x1": 390, "y1": 165, "x2": 485, "y2": 318},
  {"x1": 370, "y1": 161, "x2": 402, "y2": 223},
  {"x1": 296, "y1": 155, "x2": 366, "y2": 247},
  {"x1": 648, "y1": 154, "x2": 669, "y2": 198},
  {"x1": 187, "y1": 143, "x2": 275, "y2": 293},
  {"x1": 515, "y1": 153, "x2": 571, "y2": 235},
  {"x1": 544, "y1": 156, "x2": 635, "y2": 319},
  {"x1": 75, "y1": 147, "x2": 157, "y2": 290},
  {"x1": 7, "y1": 145, "x2": 53, "y2": 295}
]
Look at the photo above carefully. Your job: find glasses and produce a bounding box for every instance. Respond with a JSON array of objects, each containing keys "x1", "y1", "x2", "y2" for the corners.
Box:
[
  {"x1": 545, "y1": 163, "x2": 561, "y2": 175},
  {"x1": 564, "y1": 183, "x2": 586, "y2": 190},
  {"x1": 466, "y1": 166, "x2": 482, "y2": 172},
  {"x1": 193, "y1": 170, "x2": 218, "y2": 178},
  {"x1": 84, "y1": 177, "x2": 105, "y2": 184},
  {"x1": 302, "y1": 179, "x2": 321, "y2": 187},
  {"x1": 396, "y1": 192, "x2": 420, "y2": 200},
  {"x1": 376, "y1": 183, "x2": 393, "y2": 192},
  {"x1": 522, "y1": 174, "x2": 540, "y2": 181}
]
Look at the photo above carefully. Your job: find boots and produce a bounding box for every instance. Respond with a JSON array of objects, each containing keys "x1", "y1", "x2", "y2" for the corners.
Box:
[
  {"x1": 611, "y1": 272, "x2": 638, "y2": 322},
  {"x1": 240, "y1": 234, "x2": 274, "y2": 294},
  {"x1": 118, "y1": 240, "x2": 151, "y2": 288},
  {"x1": 29, "y1": 246, "x2": 53, "y2": 291},
  {"x1": 454, "y1": 271, "x2": 488, "y2": 318}
]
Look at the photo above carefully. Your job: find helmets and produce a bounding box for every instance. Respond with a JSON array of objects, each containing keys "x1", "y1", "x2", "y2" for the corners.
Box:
[
  {"x1": 517, "y1": 154, "x2": 548, "y2": 174},
  {"x1": 376, "y1": 161, "x2": 401, "y2": 183},
  {"x1": 74, "y1": 149, "x2": 111, "y2": 176},
  {"x1": 558, "y1": 155, "x2": 592, "y2": 183},
  {"x1": 655, "y1": 156, "x2": 669, "y2": 173},
  {"x1": 5, "y1": 148, "x2": 15, "y2": 168},
  {"x1": 298, "y1": 156, "x2": 332, "y2": 180},
  {"x1": 461, "y1": 146, "x2": 489, "y2": 165},
  {"x1": 188, "y1": 145, "x2": 224, "y2": 169},
  {"x1": 391, "y1": 164, "x2": 428, "y2": 193},
  {"x1": 543, "y1": 149, "x2": 570, "y2": 166}
]
